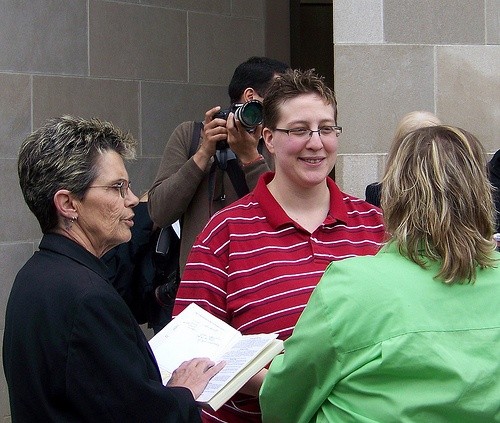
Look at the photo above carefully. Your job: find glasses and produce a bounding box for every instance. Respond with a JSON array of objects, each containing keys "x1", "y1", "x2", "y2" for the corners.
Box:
[
  {"x1": 87, "y1": 181, "x2": 132, "y2": 199},
  {"x1": 271, "y1": 125, "x2": 343, "y2": 137}
]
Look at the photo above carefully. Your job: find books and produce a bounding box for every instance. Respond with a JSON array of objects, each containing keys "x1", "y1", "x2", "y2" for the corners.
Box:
[{"x1": 147, "y1": 302, "x2": 286, "y2": 412}]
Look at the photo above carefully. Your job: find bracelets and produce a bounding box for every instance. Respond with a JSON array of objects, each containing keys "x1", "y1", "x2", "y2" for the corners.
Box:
[{"x1": 243, "y1": 154, "x2": 264, "y2": 167}]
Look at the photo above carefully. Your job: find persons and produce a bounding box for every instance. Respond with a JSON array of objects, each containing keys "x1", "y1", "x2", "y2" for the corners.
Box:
[
  {"x1": 256, "y1": 123, "x2": 499, "y2": 423},
  {"x1": 148, "y1": 56, "x2": 292, "y2": 285},
  {"x1": 96, "y1": 186, "x2": 182, "y2": 342},
  {"x1": 365, "y1": 109, "x2": 444, "y2": 209},
  {"x1": 1, "y1": 115, "x2": 228, "y2": 422},
  {"x1": 172, "y1": 69, "x2": 392, "y2": 423}
]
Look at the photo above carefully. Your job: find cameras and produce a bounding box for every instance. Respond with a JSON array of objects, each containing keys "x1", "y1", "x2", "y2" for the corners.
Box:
[
  {"x1": 155, "y1": 269, "x2": 180, "y2": 310},
  {"x1": 211, "y1": 99, "x2": 264, "y2": 150}
]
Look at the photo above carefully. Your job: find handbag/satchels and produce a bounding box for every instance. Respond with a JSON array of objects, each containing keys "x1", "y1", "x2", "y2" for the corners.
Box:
[{"x1": 156, "y1": 224, "x2": 180, "y2": 261}]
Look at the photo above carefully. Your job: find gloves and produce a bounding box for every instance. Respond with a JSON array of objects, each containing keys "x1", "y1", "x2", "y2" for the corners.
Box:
[{"x1": 156, "y1": 270, "x2": 181, "y2": 306}]
